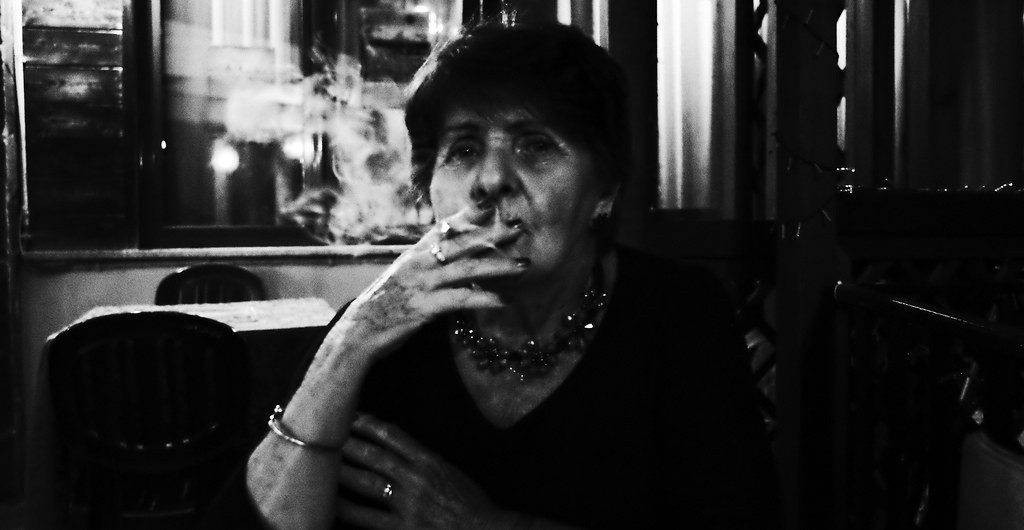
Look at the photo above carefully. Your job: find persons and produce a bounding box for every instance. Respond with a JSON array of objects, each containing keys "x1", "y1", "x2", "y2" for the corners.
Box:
[{"x1": 246, "y1": 18, "x2": 786, "y2": 530}]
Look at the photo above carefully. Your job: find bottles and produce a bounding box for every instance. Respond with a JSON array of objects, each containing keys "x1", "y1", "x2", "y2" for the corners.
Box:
[{"x1": 304, "y1": 133, "x2": 344, "y2": 212}]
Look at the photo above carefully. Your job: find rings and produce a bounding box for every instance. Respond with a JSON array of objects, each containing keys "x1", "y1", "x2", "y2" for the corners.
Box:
[
  {"x1": 438, "y1": 217, "x2": 454, "y2": 239},
  {"x1": 380, "y1": 480, "x2": 394, "y2": 505},
  {"x1": 431, "y1": 246, "x2": 448, "y2": 265}
]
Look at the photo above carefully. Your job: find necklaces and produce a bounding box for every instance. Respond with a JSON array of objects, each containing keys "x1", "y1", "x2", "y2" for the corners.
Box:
[{"x1": 448, "y1": 254, "x2": 608, "y2": 383}]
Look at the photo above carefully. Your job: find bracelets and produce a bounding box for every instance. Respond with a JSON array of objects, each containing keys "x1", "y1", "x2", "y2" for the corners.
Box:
[{"x1": 268, "y1": 404, "x2": 343, "y2": 453}]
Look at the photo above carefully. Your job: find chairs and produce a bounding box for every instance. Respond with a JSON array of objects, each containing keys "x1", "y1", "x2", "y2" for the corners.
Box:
[
  {"x1": 48, "y1": 313, "x2": 256, "y2": 530},
  {"x1": 155, "y1": 264, "x2": 264, "y2": 306}
]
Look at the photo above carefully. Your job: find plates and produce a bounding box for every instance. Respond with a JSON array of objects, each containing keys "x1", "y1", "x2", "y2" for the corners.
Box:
[{"x1": 163, "y1": 224, "x2": 306, "y2": 247}]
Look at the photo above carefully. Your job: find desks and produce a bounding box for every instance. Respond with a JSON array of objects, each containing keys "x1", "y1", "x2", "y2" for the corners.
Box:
[{"x1": 46, "y1": 298, "x2": 338, "y2": 399}]
